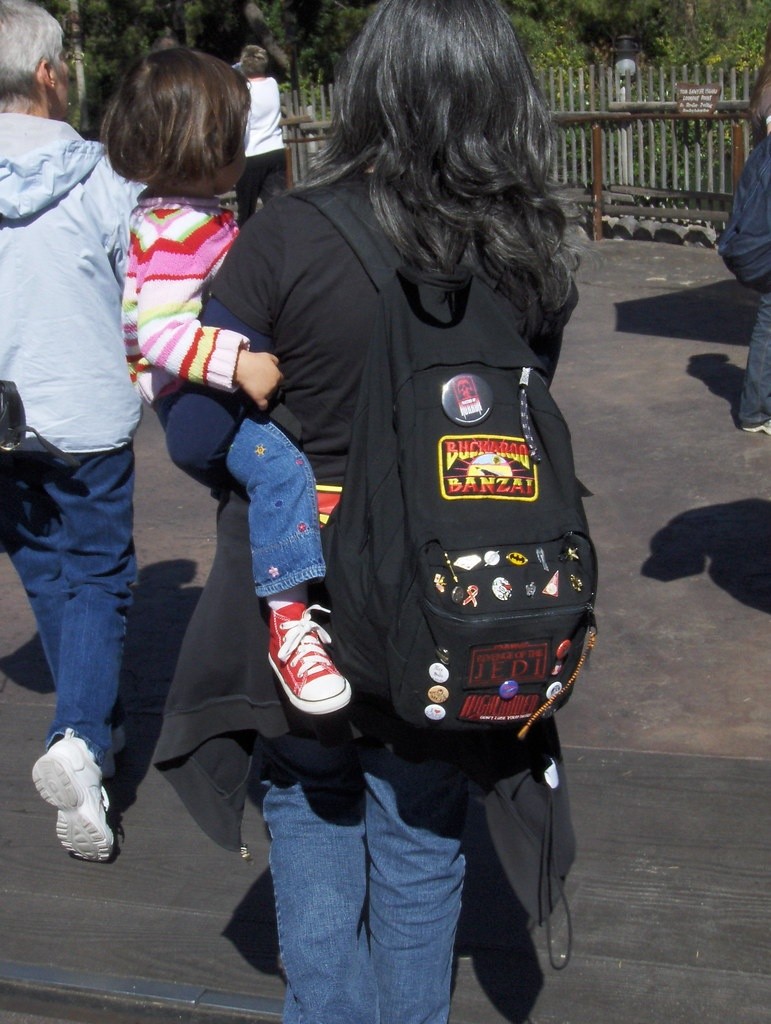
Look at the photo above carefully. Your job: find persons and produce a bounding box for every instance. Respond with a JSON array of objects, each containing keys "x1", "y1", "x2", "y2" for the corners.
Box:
[
  {"x1": 101, "y1": 48, "x2": 351, "y2": 715},
  {"x1": 738, "y1": 16, "x2": 771, "y2": 436},
  {"x1": 235, "y1": 44, "x2": 291, "y2": 231},
  {"x1": 0, "y1": 0, "x2": 147, "y2": 864},
  {"x1": 153, "y1": 0, "x2": 589, "y2": 1024}
]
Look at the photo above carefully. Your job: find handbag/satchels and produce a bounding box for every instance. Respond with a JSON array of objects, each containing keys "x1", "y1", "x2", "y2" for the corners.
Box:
[
  {"x1": 0, "y1": 379, "x2": 82, "y2": 553},
  {"x1": 716, "y1": 131, "x2": 771, "y2": 292}
]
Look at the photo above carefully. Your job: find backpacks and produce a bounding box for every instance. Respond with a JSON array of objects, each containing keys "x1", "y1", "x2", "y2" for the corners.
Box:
[{"x1": 304, "y1": 179, "x2": 598, "y2": 739}]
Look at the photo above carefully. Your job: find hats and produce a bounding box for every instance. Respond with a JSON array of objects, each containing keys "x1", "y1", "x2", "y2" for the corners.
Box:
[{"x1": 242, "y1": 44, "x2": 269, "y2": 63}]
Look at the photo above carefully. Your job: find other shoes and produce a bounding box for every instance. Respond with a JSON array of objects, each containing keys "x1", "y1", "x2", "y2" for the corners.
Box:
[{"x1": 741, "y1": 419, "x2": 771, "y2": 435}]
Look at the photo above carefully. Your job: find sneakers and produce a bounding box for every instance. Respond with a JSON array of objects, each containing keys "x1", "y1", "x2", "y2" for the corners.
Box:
[
  {"x1": 30, "y1": 727, "x2": 116, "y2": 863},
  {"x1": 268, "y1": 602, "x2": 351, "y2": 715}
]
